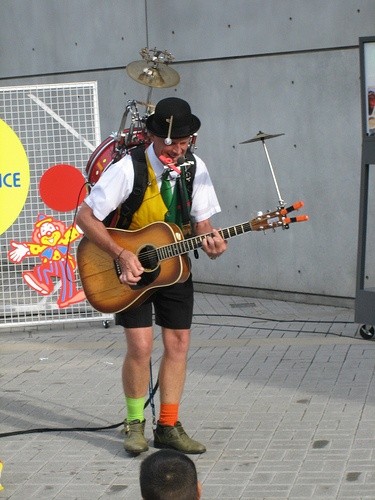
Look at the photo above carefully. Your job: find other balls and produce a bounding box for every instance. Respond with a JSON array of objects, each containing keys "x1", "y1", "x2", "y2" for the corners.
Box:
[{"x1": 39, "y1": 165, "x2": 87, "y2": 212}]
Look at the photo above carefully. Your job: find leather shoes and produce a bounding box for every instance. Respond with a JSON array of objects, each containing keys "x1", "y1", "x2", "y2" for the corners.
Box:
[
  {"x1": 153, "y1": 419, "x2": 207, "y2": 454},
  {"x1": 120, "y1": 418, "x2": 149, "y2": 454}
]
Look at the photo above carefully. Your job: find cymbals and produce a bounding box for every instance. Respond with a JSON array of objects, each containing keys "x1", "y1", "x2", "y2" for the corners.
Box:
[
  {"x1": 238, "y1": 132, "x2": 285, "y2": 144},
  {"x1": 126, "y1": 60, "x2": 180, "y2": 88}
]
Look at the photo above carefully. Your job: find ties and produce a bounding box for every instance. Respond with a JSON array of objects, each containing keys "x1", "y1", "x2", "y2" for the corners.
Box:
[{"x1": 160, "y1": 170, "x2": 172, "y2": 211}]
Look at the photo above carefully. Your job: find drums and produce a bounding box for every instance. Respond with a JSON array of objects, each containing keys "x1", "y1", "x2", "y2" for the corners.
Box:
[{"x1": 85, "y1": 127, "x2": 152, "y2": 195}]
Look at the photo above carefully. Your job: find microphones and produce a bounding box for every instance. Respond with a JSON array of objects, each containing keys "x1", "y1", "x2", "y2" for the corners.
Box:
[{"x1": 167, "y1": 156, "x2": 185, "y2": 172}]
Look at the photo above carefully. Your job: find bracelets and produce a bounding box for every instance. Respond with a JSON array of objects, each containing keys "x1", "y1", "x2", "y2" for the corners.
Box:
[{"x1": 117, "y1": 249, "x2": 125, "y2": 261}]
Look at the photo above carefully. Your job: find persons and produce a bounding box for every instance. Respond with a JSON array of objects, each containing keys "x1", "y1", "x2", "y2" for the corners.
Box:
[
  {"x1": 139, "y1": 449, "x2": 203, "y2": 500},
  {"x1": 75, "y1": 97, "x2": 228, "y2": 454}
]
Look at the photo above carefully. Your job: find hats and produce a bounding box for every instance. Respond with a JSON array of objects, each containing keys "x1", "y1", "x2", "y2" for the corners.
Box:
[{"x1": 146, "y1": 97, "x2": 201, "y2": 138}]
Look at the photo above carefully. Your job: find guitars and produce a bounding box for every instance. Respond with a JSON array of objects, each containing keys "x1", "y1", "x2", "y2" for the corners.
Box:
[{"x1": 76, "y1": 205, "x2": 283, "y2": 314}]
